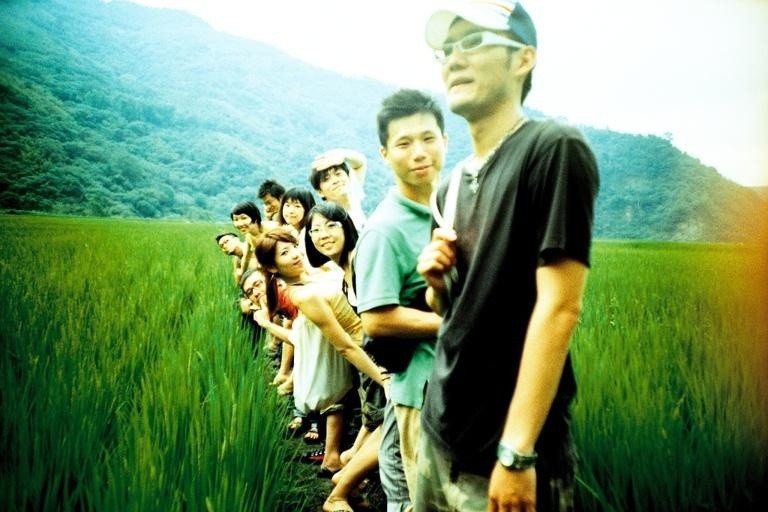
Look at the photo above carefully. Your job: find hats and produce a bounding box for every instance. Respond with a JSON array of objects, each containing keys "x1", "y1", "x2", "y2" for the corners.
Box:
[{"x1": 425, "y1": 0, "x2": 537, "y2": 50}]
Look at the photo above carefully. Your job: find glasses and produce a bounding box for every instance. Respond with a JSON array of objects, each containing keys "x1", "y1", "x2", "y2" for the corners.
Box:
[
  {"x1": 307, "y1": 222, "x2": 340, "y2": 235},
  {"x1": 244, "y1": 280, "x2": 264, "y2": 296},
  {"x1": 434, "y1": 32, "x2": 525, "y2": 60}
]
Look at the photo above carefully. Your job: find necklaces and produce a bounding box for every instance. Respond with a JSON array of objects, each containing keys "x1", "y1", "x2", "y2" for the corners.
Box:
[{"x1": 469, "y1": 115, "x2": 524, "y2": 194}]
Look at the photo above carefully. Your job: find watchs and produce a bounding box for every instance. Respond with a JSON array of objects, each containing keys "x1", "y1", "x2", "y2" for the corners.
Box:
[{"x1": 497, "y1": 441, "x2": 539, "y2": 471}]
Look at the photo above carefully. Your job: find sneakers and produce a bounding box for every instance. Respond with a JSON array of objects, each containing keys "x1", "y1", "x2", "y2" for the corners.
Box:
[{"x1": 300, "y1": 450, "x2": 324, "y2": 464}]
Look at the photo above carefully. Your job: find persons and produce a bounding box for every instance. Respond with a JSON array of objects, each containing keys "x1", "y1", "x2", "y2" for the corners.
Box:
[
  {"x1": 303, "y1": 205, "x2": 389, "y2": 435},
  {"x1": 309, "y1": 149, "x2": 367, "y2": 238},
  {"x1": 255, "y1": 228, "x2": 393, "y2": 511},
  {"x1": 353, "y1": 91, "x2": 446, "y2": 510},
  {"x1": 217, "y1": 179, "x2": 360, "y2": 472},
  {"x1": 409, "y1": 0, "x2": 602, "y2": 511}
]
